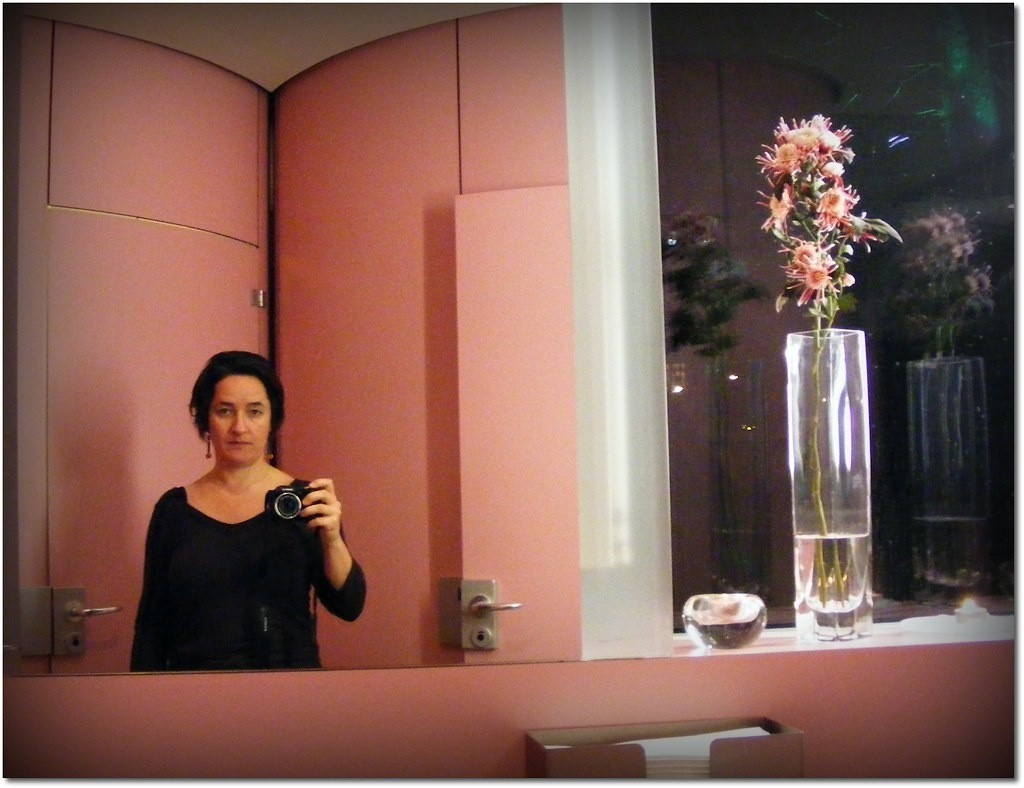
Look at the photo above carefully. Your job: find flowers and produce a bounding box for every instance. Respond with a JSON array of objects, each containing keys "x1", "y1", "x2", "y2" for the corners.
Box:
[
  {"x1": 755, "y1": 112, "x2": 902, "y2": 606},
  {"x1": 907, "y1": 211, "x2": 993, "y2": 473},
  {"x1": 663, "y1": 212, "x2": 770, "y2": 559}
]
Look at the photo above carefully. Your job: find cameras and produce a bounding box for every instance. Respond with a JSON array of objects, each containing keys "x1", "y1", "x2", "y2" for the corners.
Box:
[{"x1": 264, "y1": 479, "x2": 322, "y2": 525}]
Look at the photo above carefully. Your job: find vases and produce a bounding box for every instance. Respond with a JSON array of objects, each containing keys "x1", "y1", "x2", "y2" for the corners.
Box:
[
  {"x1": 784, "y1": 330, "x2": 873, "y2": 641},
  {"x1": 907, "y1": 356, "x2": 988, "y2": 603}
]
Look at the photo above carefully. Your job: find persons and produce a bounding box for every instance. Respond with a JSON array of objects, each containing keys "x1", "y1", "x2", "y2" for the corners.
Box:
[{"x1": 128, "y1": 351, "x2": 368, "y2": 672}]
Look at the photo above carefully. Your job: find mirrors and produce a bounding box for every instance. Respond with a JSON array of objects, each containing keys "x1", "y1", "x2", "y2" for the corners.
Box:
[{"x1": 3, "y1": 2, "x2": 1016, "y2": 674}]
